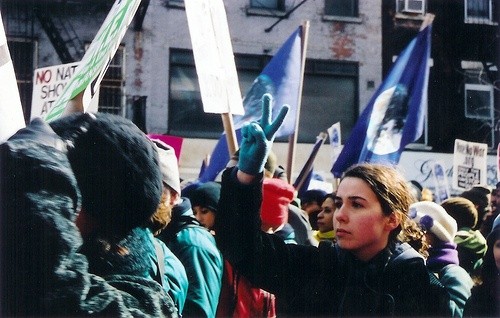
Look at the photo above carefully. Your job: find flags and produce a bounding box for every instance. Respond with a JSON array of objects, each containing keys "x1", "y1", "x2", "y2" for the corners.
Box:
[
  {"x1": 331, "y1": 23, "x2": 430, "y2": 178},
  {"x1": 198, "y1": 26, "x2": 302, "y2": 184}
]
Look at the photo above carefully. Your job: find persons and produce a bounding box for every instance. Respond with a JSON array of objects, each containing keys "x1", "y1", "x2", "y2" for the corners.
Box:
[{"x1": 0, "y1": 94, "x2": 500, "y2": 318}]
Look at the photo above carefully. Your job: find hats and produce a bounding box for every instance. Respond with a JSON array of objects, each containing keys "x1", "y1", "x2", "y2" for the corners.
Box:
[
  {"x1": 299, "y1": 188, "x2": 327, "y2": 204},
  {"x1": 260, "y1": 177, "x2": 295, "y2": 227},
  {"x1": 406, "y1": 179, "x2": 423, "y2": 198},
  {"x1": 407, "y1": 200, "x2": 458, "y2": 243},
  {"x1": 190, "y1": 181, "x2": 221, "y2": 212},
  {"x1": 50, "y1": 110, "x2": 164, "y2": 243},
  {"x1": 485, "y1": 213, "x2": 500, "y2": 246},
  {"x1": 150, "y1": 138, "x2": 182, "y2": 197}
]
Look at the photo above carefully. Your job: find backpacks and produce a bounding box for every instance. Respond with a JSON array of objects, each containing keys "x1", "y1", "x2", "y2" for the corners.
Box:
[{"x1": 167, "y1": 215, "x2": 275, "y2": 318}]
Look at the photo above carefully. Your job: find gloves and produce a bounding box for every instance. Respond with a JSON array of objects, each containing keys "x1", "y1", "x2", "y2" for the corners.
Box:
[
  {"x1": 7, "y1": 111, "x2": 98, "y2": 156},
  {"x1": 235, "y1": 92, "x2": 290, "y2": 176}
]
